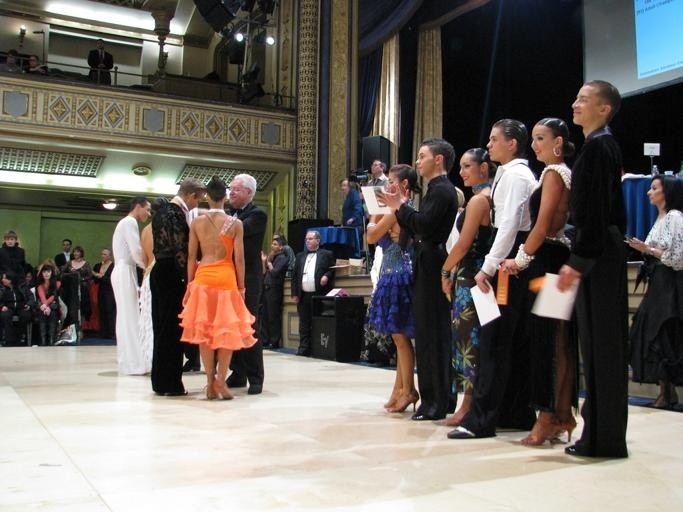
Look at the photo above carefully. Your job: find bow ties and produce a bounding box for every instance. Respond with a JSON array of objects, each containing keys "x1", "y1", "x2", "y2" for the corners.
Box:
[{"x1": 229, "y1": 208, "x2": 242, "y2": 216}]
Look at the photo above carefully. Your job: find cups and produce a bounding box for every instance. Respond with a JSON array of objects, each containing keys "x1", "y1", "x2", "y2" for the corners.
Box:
[{"x1": 664, "y1": 170, "x2": 673, "y2": 176}]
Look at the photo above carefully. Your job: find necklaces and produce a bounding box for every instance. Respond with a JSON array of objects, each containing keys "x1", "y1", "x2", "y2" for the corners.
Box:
[{"x1": 471, "y1": 182, "x2": 491, "y2": 193}]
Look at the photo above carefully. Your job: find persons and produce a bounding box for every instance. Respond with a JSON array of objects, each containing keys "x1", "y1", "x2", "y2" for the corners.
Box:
[
  {"x1": 441, "y1": 148, "x2": 497, "y2": 426},
  {"x1": 88, "y1": 40, "x2": 113, "y2": 84},
  {"x1": 500, "y1": 117, "x2": 579, "y2": 445},
  {"x1": 365, "y1": 164, "x2": 423, "y2": 413},
  {"x1": 627, "y1": 175, "x2": 683, "y2": 410},
  {"x1": 375, "y1": 139, "x2": 459, "y2": 421},
  {"x1": 447, "y1": 119, "x2": 538, "y2": 438},
  {"x1": 0, "y1": 49, "x2": 22, "y2": 74},
  {"x1": 24, "y1": 55, "x2": 48, "y2": 76},
  {"x1": 557, "y1": 81, "x2": 627, "y2": 458}
]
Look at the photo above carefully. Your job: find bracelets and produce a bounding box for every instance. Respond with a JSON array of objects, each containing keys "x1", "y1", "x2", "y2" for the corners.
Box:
[
  {"x1": 650, "y1": 248, "x2": 655, "y2": 256},
  {"x1": 514, "y1": 244, "x2": 536, "y2": 270},
  {"x1": 441, "y1": 270, "x2": 457, "y2": 280}
]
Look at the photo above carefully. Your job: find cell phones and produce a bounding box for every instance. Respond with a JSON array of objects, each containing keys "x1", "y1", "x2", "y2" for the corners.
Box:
[{"x1": 624, "y1": 233, "x2": 633, "y2": 241}]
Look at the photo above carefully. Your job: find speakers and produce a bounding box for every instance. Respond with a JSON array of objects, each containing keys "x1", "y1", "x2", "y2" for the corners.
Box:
[
  {"x1": 229, "y1": 42, "x2": 244, "y2": 64},
  {"x1": 312, "y1": 294, "x2": 365, "y2": 363},
  {"x1": 362, "y1": 136, "x2": 389, "y2": 172}
]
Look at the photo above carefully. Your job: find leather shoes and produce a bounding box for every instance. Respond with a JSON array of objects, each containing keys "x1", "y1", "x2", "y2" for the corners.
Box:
[
  {"x1": 0, "y1": 334, "x2": 58, "y2": 347},
  {"x1": 445, "y1": 428, "x2": 496, "y2": 440},
  {"x1": 247, "y1": 383, "x2": 262, "y2": 395},
  {"x1": 157, "y1": 389, "x2": 189, "y2": 396},
  {"x1": 295, "y1": 347, "x2": 310, "y2": 356},
  {"x1": 262, "y1": 342, "x2": 280, "y2": 349},
  {"x1": 181, "y1": 359, "x2": 201, "y2": 372},
  {"x1": 563, "y1": 444, "x2": 629, "y2": 459},
  {"x1": 224, "y1": 371, "x2": 247, "y2": 388},
  {"x1": 431, "y1": 409, "x2": 466, "y2": 427},
  {"x1": 409, "y1": 406, "x2": 454, "y2": 421}
]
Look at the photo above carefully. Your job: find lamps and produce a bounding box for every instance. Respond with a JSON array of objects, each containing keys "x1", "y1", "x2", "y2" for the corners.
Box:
[
  {"x1": 17, "y1": 24, "x2": 26, "y2": 48},
  {"x1": 102, "y1": 199, "x2": 119, "y2": 210},
  {"x1": 232, "y1": 29, "x2": 275, "y2": 51}
]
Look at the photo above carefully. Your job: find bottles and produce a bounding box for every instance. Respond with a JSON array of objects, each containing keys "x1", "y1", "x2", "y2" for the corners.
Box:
[{"x1": 651, "y1": 165, "x2": 659, "y2": 177}]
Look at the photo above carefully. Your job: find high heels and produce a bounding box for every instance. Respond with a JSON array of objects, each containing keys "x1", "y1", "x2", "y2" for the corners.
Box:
[
  {"x1": 641, "y1": 393, "x2": 680, "y2": 410},
  {"x1": 382, "y1": 389, "x2": 401, "y2": 409},
  {"x1": 382, "y1": 390, "x2": 420, "y2": 415},
  {"x1": 206, "y1": 385, "x2": 218, "y2": 400},
  {"x1": 558, "y1": 415, "x2": 578, "y2": 444},
  {"x1": 212, "y1": 377, "x2": 233, "y2": 401},
  {"x1": 518, "y1": 420, "x2": 562, "y2": 447}
]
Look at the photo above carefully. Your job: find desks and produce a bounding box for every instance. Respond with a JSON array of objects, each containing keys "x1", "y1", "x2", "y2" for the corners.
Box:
[
  {"x1": 303, "y1": 226, "x2": 360, "y2": 258},
  {"x1": 622, "y1": 176, "x2": 681, "y2": 242}
]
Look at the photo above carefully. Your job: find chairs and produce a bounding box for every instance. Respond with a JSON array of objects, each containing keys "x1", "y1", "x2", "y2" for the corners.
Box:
[
  {"x1": 17, "y1": 280, "x2": 58, "y2": 344},
  {"x1": 0, "y1": 283, "x2": 30, "y2": 347}
]
